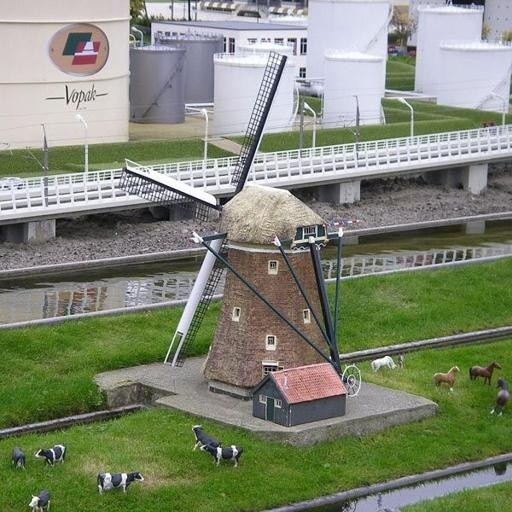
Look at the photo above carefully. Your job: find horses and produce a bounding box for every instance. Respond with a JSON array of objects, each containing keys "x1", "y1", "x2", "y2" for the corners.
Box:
[
  {"x1": 371, "y1": 355, "x2": 398, "y2": 374},
  {"x1": 432, "y1": 365, "x2": 460, "y2": 392},
  {"x1": 489, "y1": 376, "x2": 510, "y2": 417},
  {"x1": 470, "y1": 361, "x2": 502, "y2": 385}
]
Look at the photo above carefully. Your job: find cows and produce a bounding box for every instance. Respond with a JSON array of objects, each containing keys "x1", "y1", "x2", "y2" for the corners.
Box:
[
  {"x1": 191, "y1": 423, "x2": 220, "y2": 452},
  {"x1": 10, "y1": 446, "x2": 28, "y2": 472},
  {"x1": 199, "y1": 445, "x2": 244, "y2": 468},
  {"x1": 28, "y1": 489, "x2": 52, "y2": 512},
  {"x1": 34, "y1": 444, "x2": 67, "y2": 468},
  {"x1": 96, "y1": 471, "x2": 145, "y2": 496}
]
